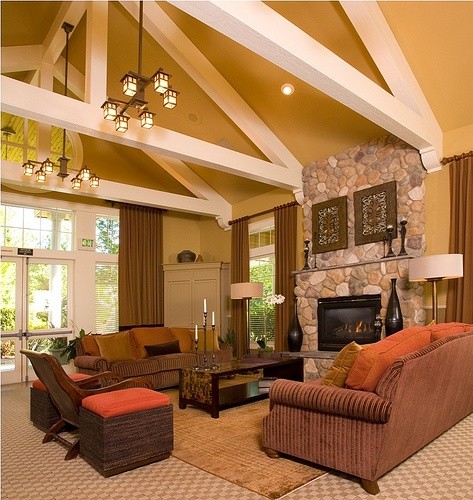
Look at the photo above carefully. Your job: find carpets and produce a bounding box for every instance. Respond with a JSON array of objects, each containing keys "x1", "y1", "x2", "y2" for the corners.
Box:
[{"x1": 162, "y1": 374, "x2": 331, "y2": 499}]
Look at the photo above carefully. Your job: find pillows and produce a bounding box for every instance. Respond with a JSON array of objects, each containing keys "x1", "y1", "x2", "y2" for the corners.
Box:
[
  {"x1": 320, "y1": 340, "x2": 363, "y2": 388},
  {"x1": 94, "y1": 331, "x2": 181, "y2": 362},
  {"x1": 188, "y1": 329, "x2": 218, "y2": 351}
]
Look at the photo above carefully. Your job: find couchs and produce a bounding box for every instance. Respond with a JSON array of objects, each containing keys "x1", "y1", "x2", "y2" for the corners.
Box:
[
  {"x1": 73, "y1": 326, "x2": 206, "y2": 388},
  {"x1": 261, "y1": 321, "x2": 473, "y2": 496}
]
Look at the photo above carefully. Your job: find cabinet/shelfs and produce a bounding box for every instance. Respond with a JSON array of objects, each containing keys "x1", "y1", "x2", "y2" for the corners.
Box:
[{"x1": 161, "y1": 261, "x2": 229, "y2": 338}]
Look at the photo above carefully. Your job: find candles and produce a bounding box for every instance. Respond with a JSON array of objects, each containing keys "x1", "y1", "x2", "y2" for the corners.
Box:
[{"x1": 194, "y1": 299, "x2": 216, "y2": 337}]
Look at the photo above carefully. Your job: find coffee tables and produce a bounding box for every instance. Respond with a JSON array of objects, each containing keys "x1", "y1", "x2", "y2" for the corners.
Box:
[{"x1": 179, "y1": 357, "x2": 303, "y2": 418}]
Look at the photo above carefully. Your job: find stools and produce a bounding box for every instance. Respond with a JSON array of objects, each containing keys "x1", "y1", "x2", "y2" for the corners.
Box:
[{"x1": 29, "y1": 372, "x2": 174, "y2": 477}]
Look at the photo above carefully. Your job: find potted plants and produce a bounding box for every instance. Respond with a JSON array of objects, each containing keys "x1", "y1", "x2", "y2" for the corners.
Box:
[
  {"x1": 258, "y1": 339, "x2": 273, "y2": 358},
  {"x1": 47, "y1": 323, "x2": 68, "y2": 364},
  {"x1": 216, "y1": 329, "x2": 236, "y2": 356}
]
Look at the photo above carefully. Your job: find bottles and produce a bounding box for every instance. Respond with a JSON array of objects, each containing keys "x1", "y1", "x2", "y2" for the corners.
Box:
[
  {"x1": 385, "y1": 278, "x2": 403, "y2": 338},
  {"x1": 288, "y1": 299, "x2": 303, "y2": 352}
]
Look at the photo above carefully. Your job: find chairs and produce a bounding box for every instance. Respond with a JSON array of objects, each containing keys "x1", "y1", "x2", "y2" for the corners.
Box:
[{"x1": 20, "y1": 349, "x2": 156, "y2": 461}]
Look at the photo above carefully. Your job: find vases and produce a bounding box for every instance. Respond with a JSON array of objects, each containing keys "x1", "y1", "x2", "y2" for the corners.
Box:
[
  {"x1": 385, "y1": 278, "x2": 403, "y2": 337},
  {"x1": 287, "y1": 298, "x2": 303, "y2": 352}
]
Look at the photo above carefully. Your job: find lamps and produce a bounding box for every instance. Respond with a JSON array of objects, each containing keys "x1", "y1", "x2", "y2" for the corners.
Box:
[
  {"x1": 407, "y1": 253, "x2": 464, "y2": 324},
  {"x1": 20, "y1": 21, "x2": 101, "y2": 191},
  {"x1": 231, "y1": 282, "x2": 265, "y2": 361},
  {"x1": 100, "y1": 0, "x2": 180, "y2": 134}
]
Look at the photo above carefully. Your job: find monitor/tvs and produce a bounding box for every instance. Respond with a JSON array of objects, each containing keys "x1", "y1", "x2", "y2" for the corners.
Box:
[{"x1": 317, "y1": 300, "x2": 381, "y2": 351}]
